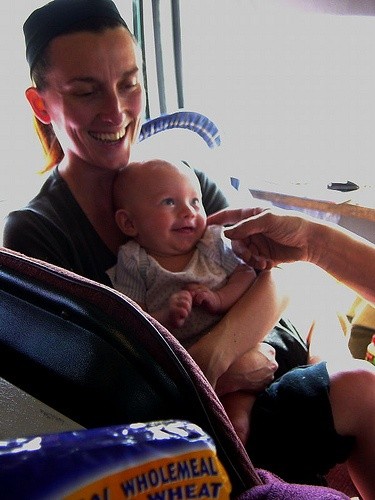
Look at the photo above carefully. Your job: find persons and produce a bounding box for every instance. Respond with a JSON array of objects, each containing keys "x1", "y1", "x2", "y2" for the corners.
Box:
[
  {"x1": 206, "y1": 203, "x2": 375, "y2": 308},
  {"x1": 3, "y1": 0, "x2": 375, "y2": 500},
  {"x1": 112, "y1": 159, "x2": 258, "y2": 448}
]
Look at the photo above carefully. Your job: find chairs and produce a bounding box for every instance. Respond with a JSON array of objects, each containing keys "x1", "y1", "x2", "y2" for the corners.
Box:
[{"x1": 0, "y1": 110, "x2": 262, "y2": 500}]
